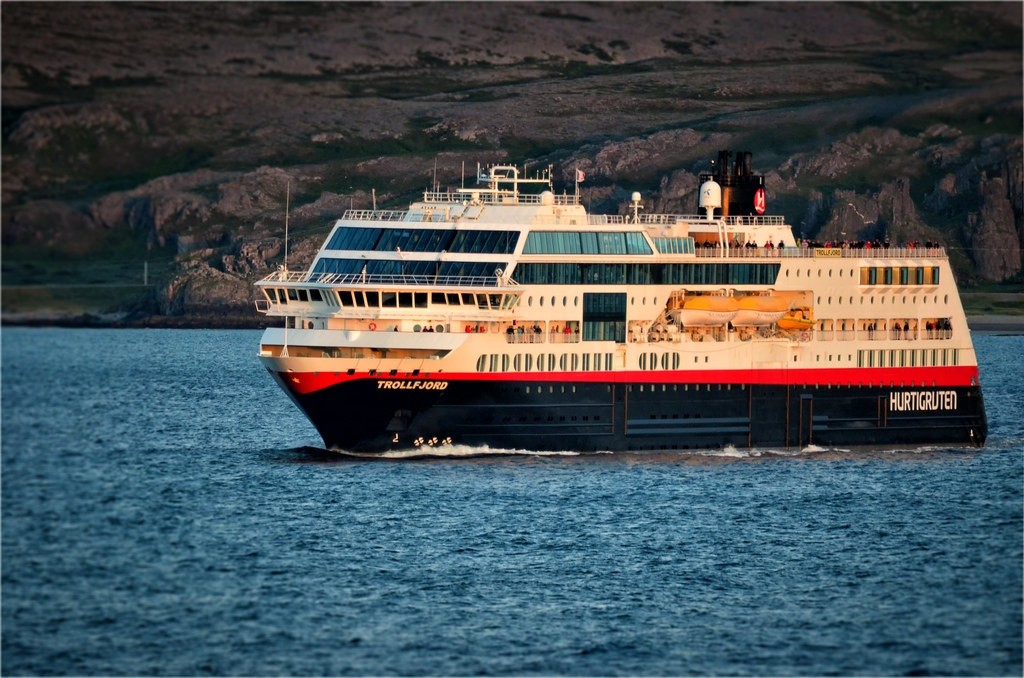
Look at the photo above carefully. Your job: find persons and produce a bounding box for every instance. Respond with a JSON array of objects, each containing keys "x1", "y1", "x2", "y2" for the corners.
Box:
[
  {"x1": 926, "y1": 321, "x2": 953, "y2": 339},
  {"x1": 894, "y1": 322, "x2": 917, "y2": 340},
  {"x1": 868, "y1": 323, "x2": 874, "y2": 340},
  {"x1": 796, "y1": 238, "x2": 940, "y2": 258},
  {"x1": 550, "y1": 325, "x2": 579, "y2": 343},
  {"x1": 465, "y1": 324, "x2": 486, "y2": 333},
  {"x1": 506, "y1": 325, "x2": 542, "y2": 343},
  {"x1": 394, "y1": 325, "x2": 398, "y2": 331},
  {"x1": 423, "y1": 326, "x2": 434, "y2": 332},
  {"x1": 695, "y1": 238, "x2": 784, "y2": 257}
]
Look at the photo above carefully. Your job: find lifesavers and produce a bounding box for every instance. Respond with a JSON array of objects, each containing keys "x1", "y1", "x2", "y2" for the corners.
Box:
[{"x1": 369, "y1": 322, "x2": 376, "y2": 331}]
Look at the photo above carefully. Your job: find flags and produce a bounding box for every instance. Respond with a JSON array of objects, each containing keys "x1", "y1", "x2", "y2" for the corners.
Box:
[{"x1": 577, "y1": 170, "x2": 585, "y2": 182}]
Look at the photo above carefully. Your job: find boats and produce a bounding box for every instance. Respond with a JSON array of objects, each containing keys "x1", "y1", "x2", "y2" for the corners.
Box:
[{"x1": 252, "y1": 147, "x2": 989, "y2": 452}]
